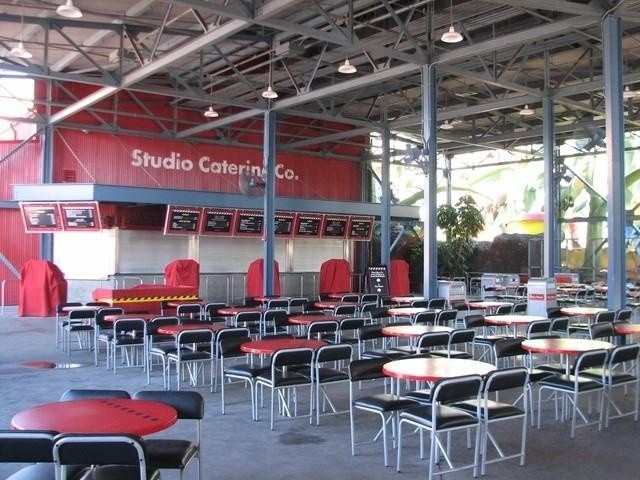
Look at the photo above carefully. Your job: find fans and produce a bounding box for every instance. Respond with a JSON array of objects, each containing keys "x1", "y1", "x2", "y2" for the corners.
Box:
[
  {"x1": 555, "y1": 165, "x2": 574, "y2": 184},
  {"x1": 573, "y1": 121, "x2": 607, "y2": 154},
  {"x1": 403, "y1": 144, "x2": 428, "y2": 175},
  {"x1": 562, "y1": 196, "x2": 575, "y2": 210},
  {"x1": 239, "y1": 168, "x2": 265, "y2": 200}
]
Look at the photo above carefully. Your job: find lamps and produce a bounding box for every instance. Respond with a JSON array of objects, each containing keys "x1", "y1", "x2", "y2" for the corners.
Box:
[
  {"x1": 10, "y1": 0, "x2": 32, "y2": 58},
  {"x1": 441, "y1": 0, "x2": 463, "y2": 42},
  {"x1": 204, "y1": 77, "x2": 219, "y2": 117},
  {"x1": 56, "y1": 0, "x2": 83, "y2": 18},
  {"x1": 263, "y1": 52, "x2": 278, "y2": 98},
  {"x1": 623, "y1": 86, "x2": 635, "y2": 98},
  {"x1": 519, "y1": 73, "x2": 534, "y2": 115},
  {"x1": 440, "y1": 95, "x2": 453, "y2": 129},
  {"x1": 337, "y1": 48, "x2": 357, "y2": 73}
]
